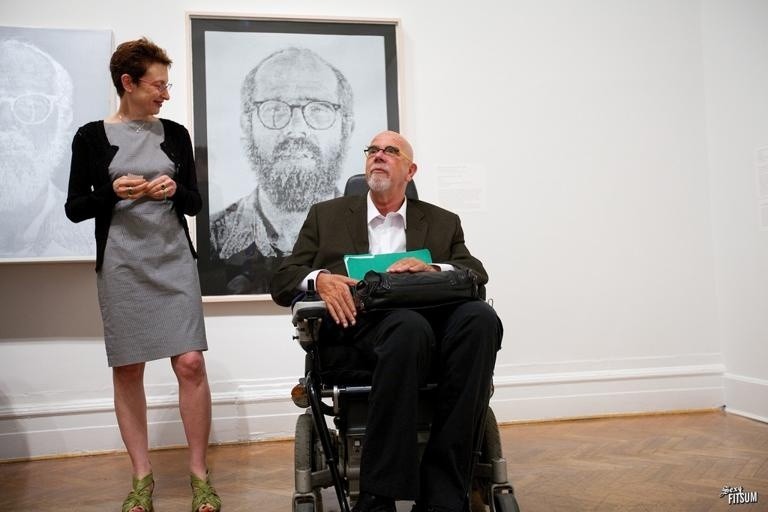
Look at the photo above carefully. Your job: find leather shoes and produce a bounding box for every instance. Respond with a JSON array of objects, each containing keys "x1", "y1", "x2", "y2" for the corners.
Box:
[{"x1": 352, "y1": 496, "x2": 397, "y2": 512}]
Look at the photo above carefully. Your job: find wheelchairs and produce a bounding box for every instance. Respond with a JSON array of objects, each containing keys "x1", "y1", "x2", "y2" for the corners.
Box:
[{"x1": 291, "y1": 172, "x2": 521, "y2": 512}]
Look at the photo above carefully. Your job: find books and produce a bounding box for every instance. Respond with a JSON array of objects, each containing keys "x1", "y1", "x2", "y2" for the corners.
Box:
[{"x1": 342, "y1": 248, "x2": 433, "y2": 281}]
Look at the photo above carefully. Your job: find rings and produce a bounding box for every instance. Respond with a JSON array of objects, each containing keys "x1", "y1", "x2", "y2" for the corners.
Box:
[
  {"x1": 126, "y1": 185, "x2": 133, "y2": 194},
  {"x1": 160, "y1": 184, "x2": 166, "y2": 189}
]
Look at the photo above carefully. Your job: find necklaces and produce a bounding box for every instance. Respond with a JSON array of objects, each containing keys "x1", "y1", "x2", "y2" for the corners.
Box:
[{"x1": 118, "y1": 112, "x2": 155, "y2": 132}]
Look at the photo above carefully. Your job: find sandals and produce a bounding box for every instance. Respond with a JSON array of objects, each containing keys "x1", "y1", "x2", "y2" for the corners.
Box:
[
  {"x1": 122, "y1": 472, "x2": 155, "y2": 512},
  {"x1": 189, "y1": 469, "x2": 221, "y2": 512}
]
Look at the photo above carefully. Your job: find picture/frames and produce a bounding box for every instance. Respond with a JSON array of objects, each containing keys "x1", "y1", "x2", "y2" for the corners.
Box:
[{"x1": 182, "y1": 11, "x2": 410, "y2": 305}]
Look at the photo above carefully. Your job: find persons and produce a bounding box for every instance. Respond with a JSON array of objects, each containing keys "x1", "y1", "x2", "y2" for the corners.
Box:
[
  {"x1": 64, "y1": 37, "x2": 222, "y2": 511},
  {"x1": 272, "y1": 130, "x2": 503, "y2": 511},
  {"x1": 209, "y1": 48, "x2": 356, "y2": 295}
]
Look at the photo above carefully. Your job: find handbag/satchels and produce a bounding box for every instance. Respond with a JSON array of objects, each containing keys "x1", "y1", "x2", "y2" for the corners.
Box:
[{"x1": 349, "y1": 269, "x2": 479, "y2": 311}]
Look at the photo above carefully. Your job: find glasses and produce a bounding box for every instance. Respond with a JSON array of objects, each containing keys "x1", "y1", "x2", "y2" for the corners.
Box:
[
  {"x1": 363, "y1": 146, "x2": 412, "y2": 163},
  {"x1": 139, "y1": 77, "x2": 172, "y2": 91},
  {"x1": 253, "y1": 99, "x2": 340, "y2": 131}
]
